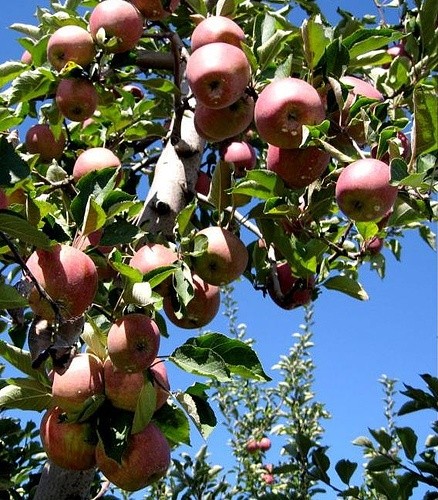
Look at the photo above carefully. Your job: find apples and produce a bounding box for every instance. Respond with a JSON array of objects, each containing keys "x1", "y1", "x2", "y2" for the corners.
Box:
[{"x1": 0, "y1": 0, "x2": 413, "y2": 492}]
